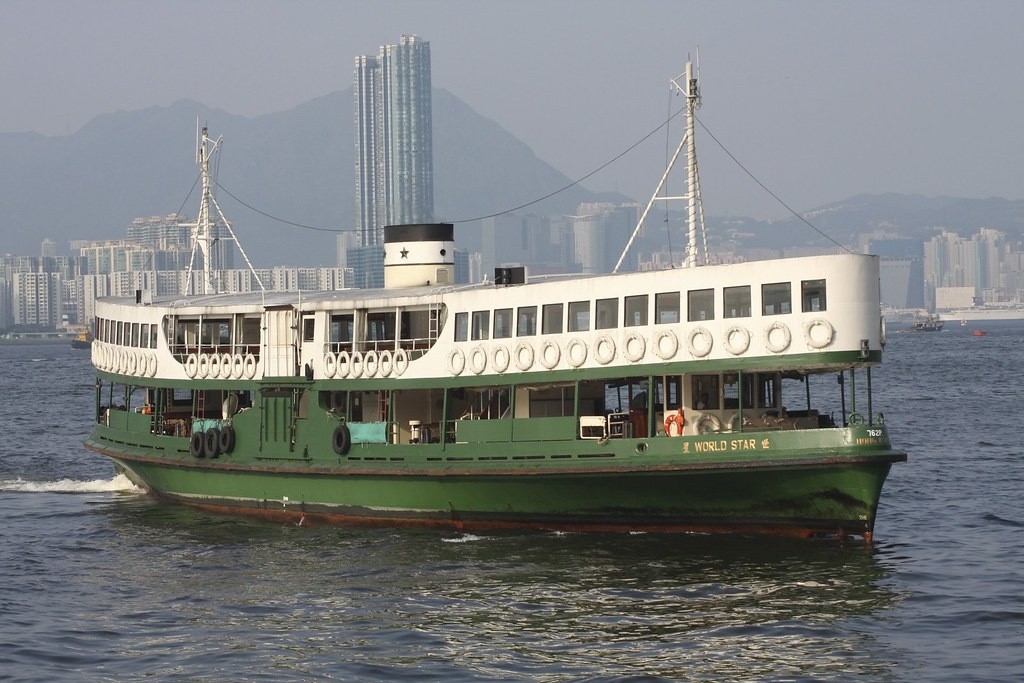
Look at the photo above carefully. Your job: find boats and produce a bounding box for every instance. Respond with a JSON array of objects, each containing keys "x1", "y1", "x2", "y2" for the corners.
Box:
[
  {"x1": 911, "y1": 309, "x2": 945, "y2": 333},
  {"x1": 81, "y1": 45, "x2": 909, "y2": 549}
]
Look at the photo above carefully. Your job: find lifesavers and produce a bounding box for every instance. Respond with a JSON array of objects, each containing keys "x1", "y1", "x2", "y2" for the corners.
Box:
[
  {"x1": 91, "y1": 341, "x2": 408, "y2": 380},
  {"x1": 467, "y1": 345, "x2": 488, "y2": 374},
  {"x1": 513, "y1": 342, "x2": 534, "y2": 371},
  {"x1": 804, "y1": 318, "x2": 832, "y2": 348},
  {"x1": 664, "y1": 411, "x2": 752, "y2": 438},
  {"x1": 654, "y1": 329, "x2": 679, "y2": 360},
  {"x1": 723, "y1": 326, "x2": 750, "y2": 355},
  {"x1": 191, "y1": 427, "x2": 234, "y2": 459},
  {"x1": 593, "y1": 336, "x2": 616, "y2": 364},
  {"x1": 333, "y1": 425, "x2": 351, "y2": 455},
  {"x1": 539, "y1": 340, "x2": 562, "y2": 368},
  {"x1": 490, "y1": 345, "x2": 509, "y2": 371},
  {"x1": 448, "y1": 347, "x2": 465, "y2": 375},
  {"x1": 565, "y1": 338, "x2": 589, "y2": 366},
  {"x1": 764, "y1": 323, "x2": 792, "y2": 352},
  {"x1": 687, "y1": 327, "x2": 713, "y2": 356},
  {"x1": 622, "y1": 332, "x2": 647, "y2": 362}
]
